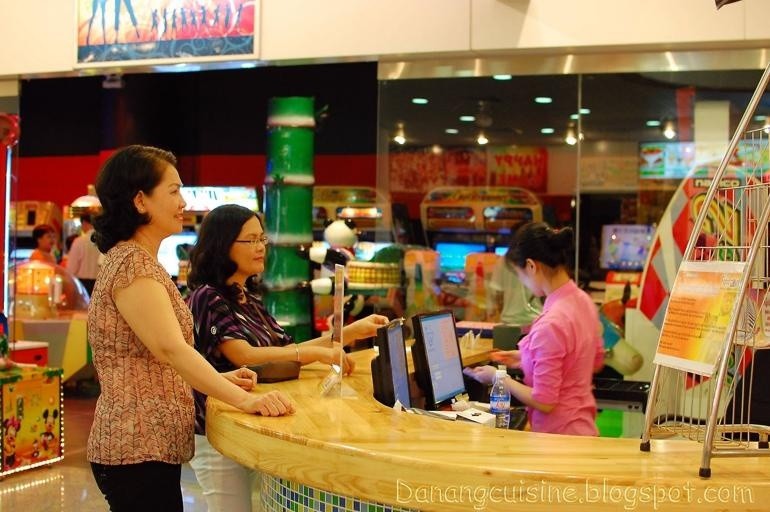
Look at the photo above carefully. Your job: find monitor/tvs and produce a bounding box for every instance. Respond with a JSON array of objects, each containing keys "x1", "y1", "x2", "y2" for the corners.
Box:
[
  {"x1": 434, "y1": 242, "x2": 487, "y2": 284},
  {"x1": 596, "y1": 221, "x2": 659, "y2": 272},
  {"x1": 157, "y1": 234, "x2": 199, "y2": 277},
  {"x1": 494, "y1": 245, "x2": 510, "y2": 257},
  {"x1": 411, "y1": 308, "x2": 470, "y2": 412},
  {"x1": 371, "y1": 318, "x2": 412, "y2": 411}
]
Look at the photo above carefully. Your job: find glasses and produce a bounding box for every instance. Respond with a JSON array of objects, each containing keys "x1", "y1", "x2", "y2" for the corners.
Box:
[{"x1": 238, "y1": 238, "x2": 269, "y2": 247}]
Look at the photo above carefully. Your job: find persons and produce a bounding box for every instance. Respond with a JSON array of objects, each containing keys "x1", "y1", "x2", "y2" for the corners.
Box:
[
  {"x1": 58, "y1": 234, "x2": 79, "y2": 267},
  {"x1": 87, "y1": 143, "x2": 296, "y2": 511},
  {"x1": 474, "y1": 219, "x2": 605, "y2": 436},
  {"x1": 66, "y1": 214, "x2": 103, "y2": 298},
  {"x1": 29, "y1": 225, "x2": 57, "y2": 264},
  {"x1": 186, "y1": 203, "x2": 390, "y2": 512},
  {"x1": 488, "y1": 222, "x2": 544, "y2": 324}
]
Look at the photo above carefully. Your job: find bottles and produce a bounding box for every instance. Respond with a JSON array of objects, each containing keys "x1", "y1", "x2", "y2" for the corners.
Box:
[{"x1": 489, "y1": 370, "x2": 511, "y2": 431}]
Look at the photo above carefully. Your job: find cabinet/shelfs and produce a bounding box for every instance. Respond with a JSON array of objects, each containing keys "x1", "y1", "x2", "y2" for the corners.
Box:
[{"x1": 640, "y1": 60, "x2": 770, "y2": 478}]
